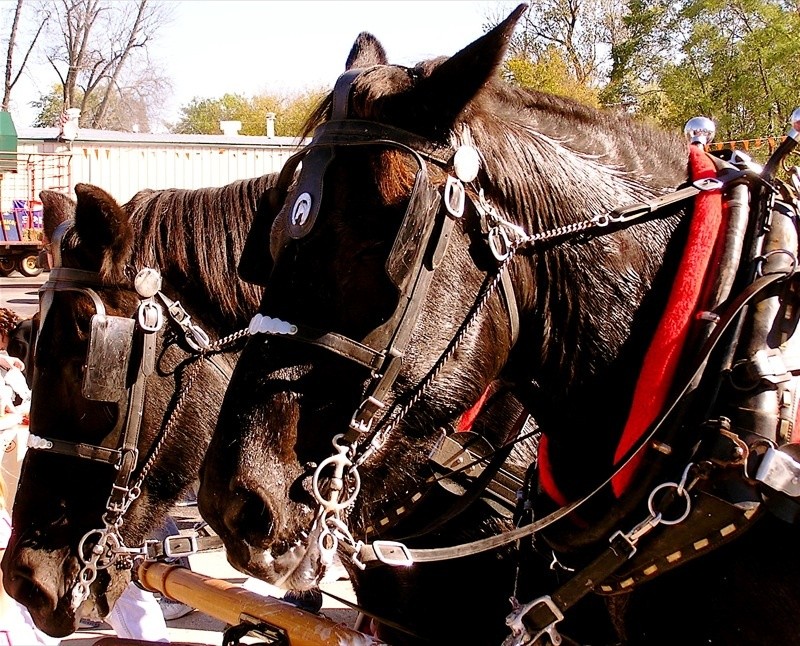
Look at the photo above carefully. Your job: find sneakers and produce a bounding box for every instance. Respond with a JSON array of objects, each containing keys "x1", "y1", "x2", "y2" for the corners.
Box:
[{"x1": 158, "y1": 596, "x2": 196, "y2": 620}]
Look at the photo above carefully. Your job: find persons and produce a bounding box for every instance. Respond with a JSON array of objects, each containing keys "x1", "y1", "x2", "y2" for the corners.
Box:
[{"x1": 0, "y1": 307, "x2": 196, "y2": 646}]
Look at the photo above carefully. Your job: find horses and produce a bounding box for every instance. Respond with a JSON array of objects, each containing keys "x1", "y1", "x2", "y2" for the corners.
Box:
[{"x1": 2, "y1": 2, "x2": 800, "y2": 646}]
